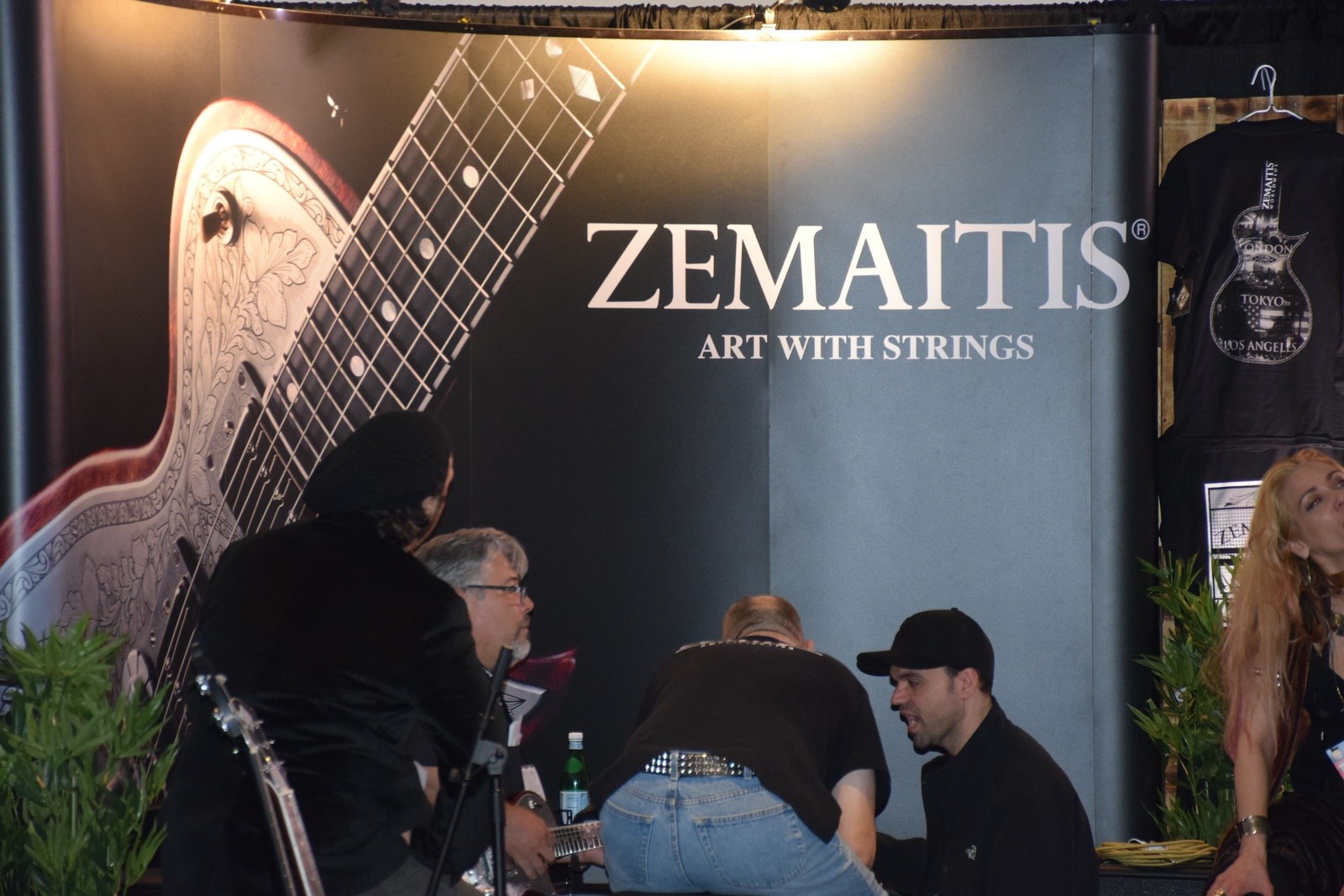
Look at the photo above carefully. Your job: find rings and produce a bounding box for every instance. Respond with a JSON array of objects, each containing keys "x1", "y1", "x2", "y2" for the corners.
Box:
[{"x1": 1209, "y1": 886, "x2": 1226, "y2": 896}]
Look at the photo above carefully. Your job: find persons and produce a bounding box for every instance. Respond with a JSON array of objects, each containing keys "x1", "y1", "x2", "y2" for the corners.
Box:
[
  {"x1": 857, "y1": 608, "x2": 1099, "y2": 896},
  {"x1": 590, "y1": 595, "x2": 891, "y2": 896},
  {"x1": 164, "y1": 410, "x2": 603, "y2": 896},
  {"x1": 1206, "y1": 446, "x2": 1344, "y2": 896}
]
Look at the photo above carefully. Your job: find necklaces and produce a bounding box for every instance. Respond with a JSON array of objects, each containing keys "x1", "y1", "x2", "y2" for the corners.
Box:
[{"x1": 1329, "y1": 611, "x2": 1344, "y2": 703}]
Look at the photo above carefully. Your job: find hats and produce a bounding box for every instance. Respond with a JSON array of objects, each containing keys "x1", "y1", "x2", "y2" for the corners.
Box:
[{"x1": 856, "y1": 607, "x2": 995, "y2": 678}]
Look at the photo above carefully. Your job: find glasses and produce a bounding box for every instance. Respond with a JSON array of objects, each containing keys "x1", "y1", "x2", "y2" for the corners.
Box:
[{"x1": 462, "y1": 582, "x2": 528, "y2": 604}]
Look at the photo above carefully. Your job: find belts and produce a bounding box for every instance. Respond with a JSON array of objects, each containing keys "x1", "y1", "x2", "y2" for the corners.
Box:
[{"x1": 640, "y1": 750, "x2": 757, "y2": 778}]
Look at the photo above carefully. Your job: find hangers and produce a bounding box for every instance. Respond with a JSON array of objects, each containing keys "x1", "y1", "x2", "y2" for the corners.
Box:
[{"x1": 1236, "y1": 65, "x2": 1304, "y2": 122}]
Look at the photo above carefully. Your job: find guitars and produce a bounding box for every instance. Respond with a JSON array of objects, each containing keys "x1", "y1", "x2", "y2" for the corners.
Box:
[
  {"x1": 464, "y1": 789, "x2": 605, "y2": 896},
  {"x1": 0, "y1": 22, "x2": 668, "y2": 809}
]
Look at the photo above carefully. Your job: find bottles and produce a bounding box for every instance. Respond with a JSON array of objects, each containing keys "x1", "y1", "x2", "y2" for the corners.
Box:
[{"x1": 559, "y1": 732, "x2": 591, "y2": 825}]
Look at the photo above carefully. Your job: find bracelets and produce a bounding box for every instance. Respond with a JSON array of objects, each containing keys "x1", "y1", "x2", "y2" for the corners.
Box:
[{"x1": 1234, "y1": 815, "x2": 1268, "y2": 841}]
